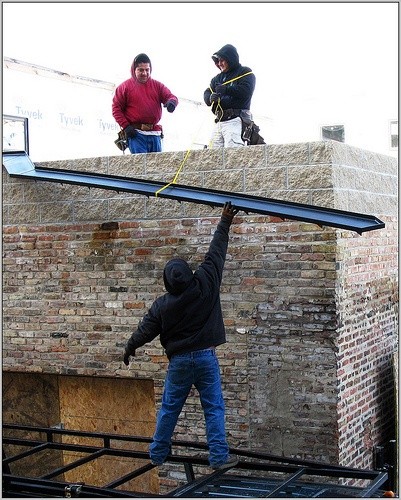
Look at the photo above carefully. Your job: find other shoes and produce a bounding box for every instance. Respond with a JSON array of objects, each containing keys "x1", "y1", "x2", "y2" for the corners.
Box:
[
  {"x1": 212, "y1": 455, "x2": 238, "y2": 469},
  {"x1": 150, "y1": 458, "x2": 163, "y2": 465}
]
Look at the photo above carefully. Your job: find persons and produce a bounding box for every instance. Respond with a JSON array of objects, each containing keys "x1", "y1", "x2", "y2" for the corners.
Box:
[
  {"x1": 204, "y1": 44, "x2": 256, "y2": 148},
  {"x1": 123, "y1": 200, "x2": 240, "y2": 471},
  {"x1": 112, "y1": 53, "x2": 179, "y2": 154}
]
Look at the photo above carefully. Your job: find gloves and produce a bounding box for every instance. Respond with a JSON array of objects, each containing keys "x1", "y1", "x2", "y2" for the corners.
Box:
[
  {"x1": 215, "y1": 83, "x2": 227, "y2": 94},
  {"x1": 124, "y1": 126, "x2": 138, "y2": 139},
  {"x1": 164, "y1": 103, "x2": 175, "y2": 113},
  {"x1": 211, "y1": 94, "x2": 218, "y2": 103},
  {"x1": 222, "y1": 200, "x2": 240, "y2": 219},
  {"x1": 123, "y1": 352, "x2": 136, "y2": 366}
]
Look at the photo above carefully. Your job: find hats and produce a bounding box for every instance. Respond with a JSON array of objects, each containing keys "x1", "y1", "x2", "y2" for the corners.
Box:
[{"x1": 212, "y1": 54, "x2": 219, "y2": 63}]
